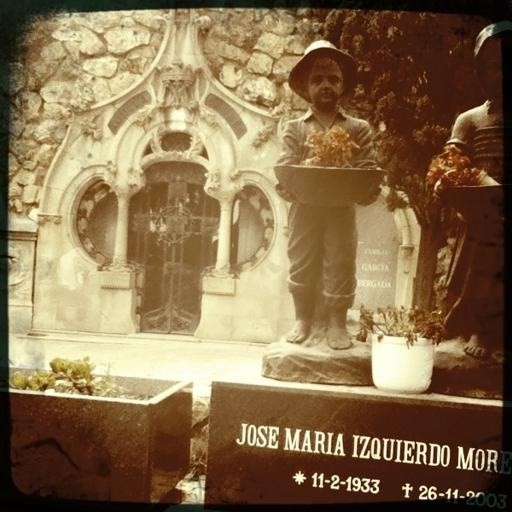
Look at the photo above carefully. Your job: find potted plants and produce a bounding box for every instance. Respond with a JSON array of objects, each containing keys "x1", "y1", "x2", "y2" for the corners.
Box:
[
  {"x1": 272, "y1": 126, "x2": 387, "y2": 209},
  {"x1": 427, "y1": 144, "x2": 504, "y2": 243},
  {"x1": 8, "y1": 358, "x2": 193, "y2": 503},
  {"x1": 352, "y1": 302, "x2": 447, "y2": 395}
]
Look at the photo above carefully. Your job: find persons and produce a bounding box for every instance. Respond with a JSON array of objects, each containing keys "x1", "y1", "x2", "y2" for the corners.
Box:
[
  {"x1": 428, "y1": 23, "x2": 505, "y2": 365},
  {"x1": 273, "y1": 40, "x2": 382, "y2": 351}
]
